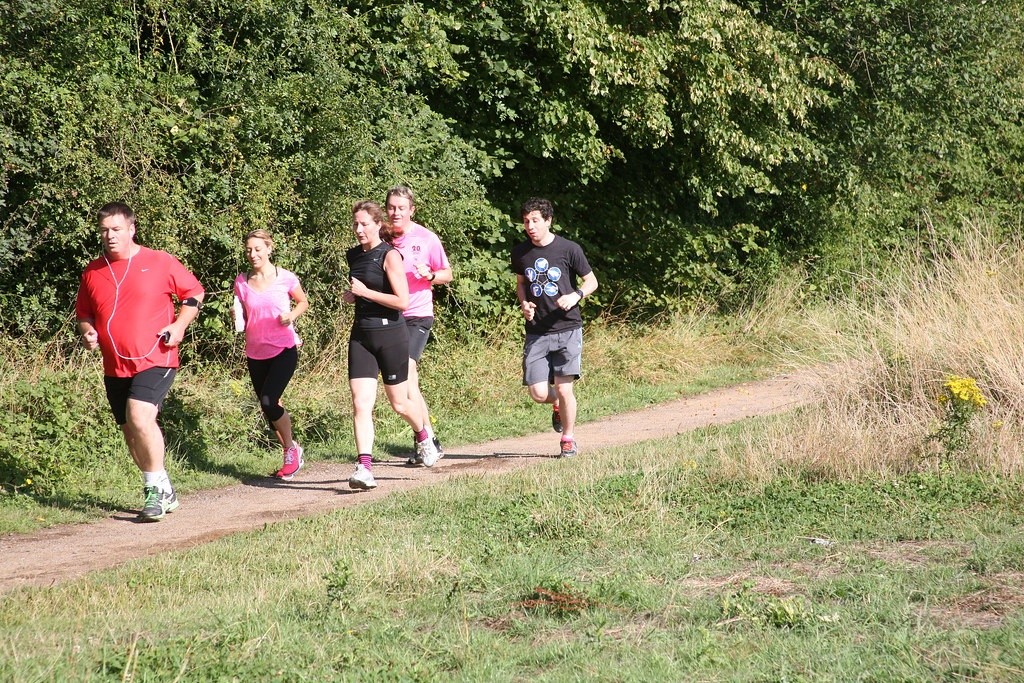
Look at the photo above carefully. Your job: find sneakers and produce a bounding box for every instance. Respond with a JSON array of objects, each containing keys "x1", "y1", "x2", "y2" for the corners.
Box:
[
  {"x1": 551, "y1": 402, "x2": 564, "y2": 432},
  {"x1": 417, "y1": 426, "x2": 437, "y2": 467},
  {"x1": 560, "y1": 436, "x2": 577, "y2": 459},
  {"x1": 349, "y1": 462, "x2": 377, "y2": 489},
  {"x1": 407, "y1": 436, "x2": 443, "y2": 464},
  {"x1": 276, "y1": 440, "x2": 304, "y2": 480},
  {"x1": 136, "y1": 485, "x2": 179, "y2": 522}
]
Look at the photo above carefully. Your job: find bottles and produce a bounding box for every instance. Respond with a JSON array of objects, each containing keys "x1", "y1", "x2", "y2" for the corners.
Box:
[{"x1": 233, "y1": 295, "x2": 244, "y2": 332}]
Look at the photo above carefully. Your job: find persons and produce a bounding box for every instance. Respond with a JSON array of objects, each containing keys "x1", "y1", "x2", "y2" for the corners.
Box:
[
  {"x1": 76, "y1": 202, "x2": 205, "y2": 522},
  {"x1": 342, "y1": 201, "x2": 437, "y2": 489},
  {"x1": 381, "y1": 186, "x2": 453, "y2": 458},
  {"x1": 234, "y1": 229, "x2": 308, "y2": 480},
  {"x1": 511, "y1": 199, "x2": 598, "y2": 457}
]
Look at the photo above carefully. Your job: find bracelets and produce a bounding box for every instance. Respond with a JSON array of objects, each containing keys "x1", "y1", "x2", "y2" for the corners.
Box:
[{"x1": 428, "y1": 273, "x2": 435, "y2": 281}]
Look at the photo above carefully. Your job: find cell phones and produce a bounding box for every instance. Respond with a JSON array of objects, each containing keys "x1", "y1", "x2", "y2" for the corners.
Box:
[{"x1": 164, "y1": 332, "x2": 171, "y2": 344}]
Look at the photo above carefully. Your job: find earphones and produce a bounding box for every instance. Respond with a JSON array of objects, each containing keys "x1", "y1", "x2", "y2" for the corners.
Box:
[{"x1": 129, "y1": 230, "x2": 133, "y2": 237}]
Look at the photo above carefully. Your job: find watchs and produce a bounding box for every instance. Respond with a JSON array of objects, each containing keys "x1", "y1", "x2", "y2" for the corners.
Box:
[{"x1": 576, "y1": 290, "x2": 583, "y2": 299}]
